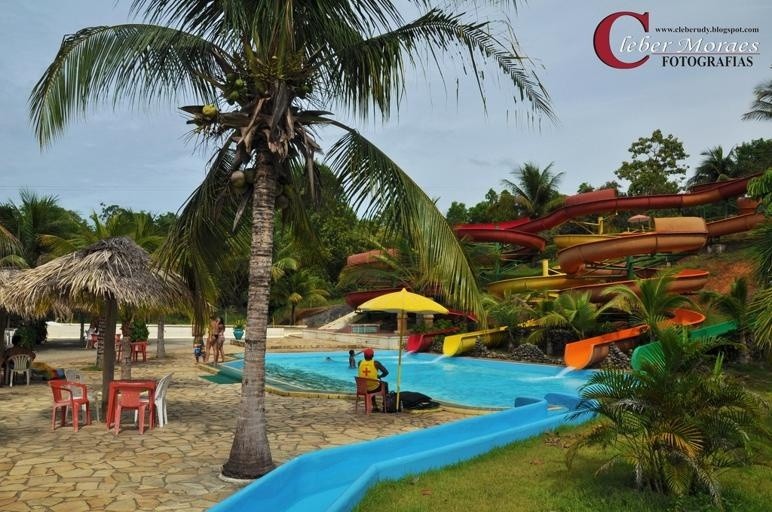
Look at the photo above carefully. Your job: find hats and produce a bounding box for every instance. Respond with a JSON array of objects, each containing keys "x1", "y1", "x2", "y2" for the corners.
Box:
[{"x1": 361, "y1": 347, "x2": 373, "y2": 355}]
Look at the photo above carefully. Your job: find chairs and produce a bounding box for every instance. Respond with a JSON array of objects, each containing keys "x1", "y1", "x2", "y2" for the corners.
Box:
[
  {"x1": 47, "y1": 378, "x2": 91, "y2": 432},
  {"x1": 105, "y1": 372, "x2": 174, "y2": 437},
  {"x1": 352, "y1": 375, "x2": 386, "y2": 415},
  {"x1": 4, "y1": 354, "x2": 32, "y2": 387}
]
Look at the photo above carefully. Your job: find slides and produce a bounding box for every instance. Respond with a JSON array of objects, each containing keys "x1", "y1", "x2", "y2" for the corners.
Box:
[{"x1": 344, "y1": 172, "x2": 772, "y2": 370}]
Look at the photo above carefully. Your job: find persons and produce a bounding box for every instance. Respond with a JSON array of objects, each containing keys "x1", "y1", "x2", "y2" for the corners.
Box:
[
  {"x1": 191, "y1": 315, "x2": 226, "y2": 365},
  {"x1": 358, "y1": 348, "x2": 390, "y2": 412},
  {"x1": 87, "y1": 320, "x2": 100, "y2": 341},
  {"x1": 632, "y1": 225, "x2": 646, "y2": 233},
  {"x1": 0, "y1": 333, "x2": 36, "y2": 369},
  {"x1": 348, "y1": 349, "x2": 355, "y2": 369}
]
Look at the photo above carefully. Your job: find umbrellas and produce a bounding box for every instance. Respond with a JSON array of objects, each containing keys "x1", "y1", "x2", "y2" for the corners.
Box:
[
  {"x1": 627, "y1": 213, "x2": 650, "y2": 233},
  {"x1": 357, "y1": 288, "x2": 450, "y2": 412}
]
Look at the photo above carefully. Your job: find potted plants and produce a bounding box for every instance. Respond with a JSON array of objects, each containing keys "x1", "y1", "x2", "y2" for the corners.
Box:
[
  {"x1": 231, "y1": 318, "x2": 245, "y2": 340},
  {"x1": 128, "y1": 318, "x2": 149, "y2": 351}
]
